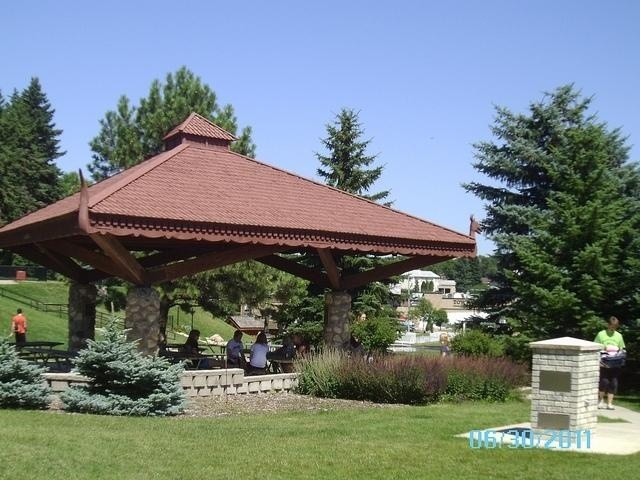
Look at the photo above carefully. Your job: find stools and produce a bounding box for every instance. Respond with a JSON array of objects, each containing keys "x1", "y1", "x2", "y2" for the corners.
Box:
[{"x1": 170, "y1": 348, "x2": 269, "y2": 375}]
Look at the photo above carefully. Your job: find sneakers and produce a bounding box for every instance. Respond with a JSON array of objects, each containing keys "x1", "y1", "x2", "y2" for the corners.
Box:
[{"x1": 598, "y1": 402, "x2": 614, "y2": 409}]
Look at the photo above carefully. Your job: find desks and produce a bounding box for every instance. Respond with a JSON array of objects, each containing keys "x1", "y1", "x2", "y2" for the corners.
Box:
[
  {"x1": 267, "y1": 358, "x2": 293, "y2": 375},
  {"x1": 10, "y1": 341, "x2": 65, "y2": 367}
]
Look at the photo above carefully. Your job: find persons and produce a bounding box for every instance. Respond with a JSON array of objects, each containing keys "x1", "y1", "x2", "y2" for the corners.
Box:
[
  {"x1": 594, "y1": 316, "x2": 626, "y2": 410},
  {"x1": 245, "y1": 333, "x2": 270, "y2": 373},
  {"x1": 288, "y1": 332, "x2": 310, "y2": 373},
  {"x1": 12, "y1": 308, "x2": 27, "y2": 345},
  {"x1": 183, "y1": 330, "x2": 212, "y2": 369},
  {"x1": 398, "y1": 311, "x2": 408, "y2": 321},
  {"x1": 227, "y1": 330, "x2": 247, "y2": 367},
  {"x1": 274, "y1": 334, "x2": 295, "y2": 356},
  {"x1": 350, "y1": 312, "x2": 366, "y2": 353},
  {"x1": 440, "y1": 332, "x2": 449, "y2": 359}
]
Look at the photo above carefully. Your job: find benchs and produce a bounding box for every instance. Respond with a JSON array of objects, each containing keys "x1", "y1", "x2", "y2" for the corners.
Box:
[{"x1": 14, "y1": 347, "x2": 76, "y2": 362}]
